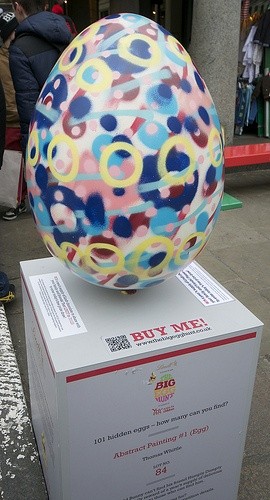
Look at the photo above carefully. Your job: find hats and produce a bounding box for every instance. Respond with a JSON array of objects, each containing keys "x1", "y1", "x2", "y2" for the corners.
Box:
[{"x1": 0, "y1": 8, "x2": 18, "y2": 43}]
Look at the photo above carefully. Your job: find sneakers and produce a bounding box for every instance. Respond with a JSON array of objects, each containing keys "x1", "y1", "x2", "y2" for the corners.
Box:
[
  {"x1": 2, "y1": 201, "x2": 27, "y2": 220},
  {"x1": 0, "y1": 284, "x2": 16, "y2": 304}
]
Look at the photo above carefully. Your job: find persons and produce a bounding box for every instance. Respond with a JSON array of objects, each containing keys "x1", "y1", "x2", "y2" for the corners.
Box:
[{"x1": 0, "y1": 1, "x2": 78, "y2": 220}]
